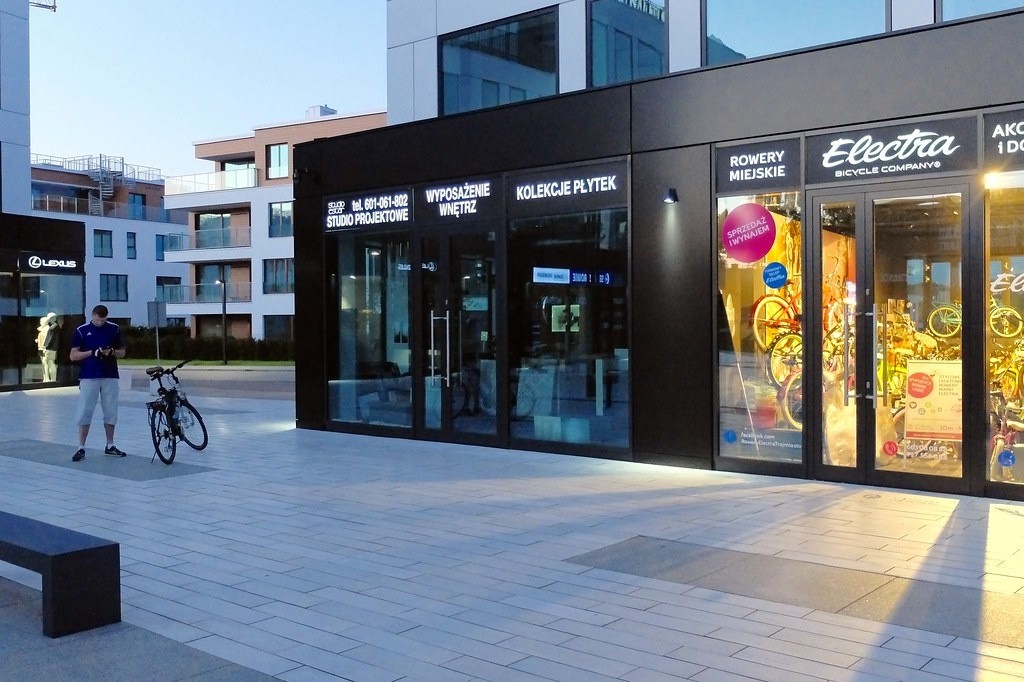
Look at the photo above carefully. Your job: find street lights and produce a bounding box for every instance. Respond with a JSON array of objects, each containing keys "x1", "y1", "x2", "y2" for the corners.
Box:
[
  {"x1": 366, "y1": 248, "x2": 381, "y2": 338},
  {"x1": 14, "y1": 251, "x2": 34, "y2": 387},
  {"x1": 216, "y1": 279, "x2": 227, "y2": 362}
]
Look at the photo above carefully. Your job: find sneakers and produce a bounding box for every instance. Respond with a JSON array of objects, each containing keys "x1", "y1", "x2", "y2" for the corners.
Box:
[
  {"x1": 104, "y1": 445, "x2": 127, "y2": 457},
  {"x1": 72, "y1": 448, "x2": 86, "y2": 461}
]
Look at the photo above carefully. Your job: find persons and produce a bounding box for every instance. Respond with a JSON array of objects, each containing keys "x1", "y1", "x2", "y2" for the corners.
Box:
[
  {"x1": 34, "y1": 312, "x2": 60, "y2": 382},
  {"x1": 69, "y1": 304, "x2": 127, "y2": 461}
]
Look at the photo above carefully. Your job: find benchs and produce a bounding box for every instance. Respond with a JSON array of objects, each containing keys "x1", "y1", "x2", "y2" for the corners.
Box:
[{"x1": 0, "y1": 510, "x2": 122, "y2": 639}]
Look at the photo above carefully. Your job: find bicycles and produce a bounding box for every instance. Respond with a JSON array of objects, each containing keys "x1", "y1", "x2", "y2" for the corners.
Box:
[
  {"x1": 144, "y1": 361, "x2": 208, "y2": 464},
  {"x1": 750, "y1": 255, "x2": 1024, "y2": 483},
  {"x1": 435, "y1": 355, "x2": 536, "y2": 422}
]
